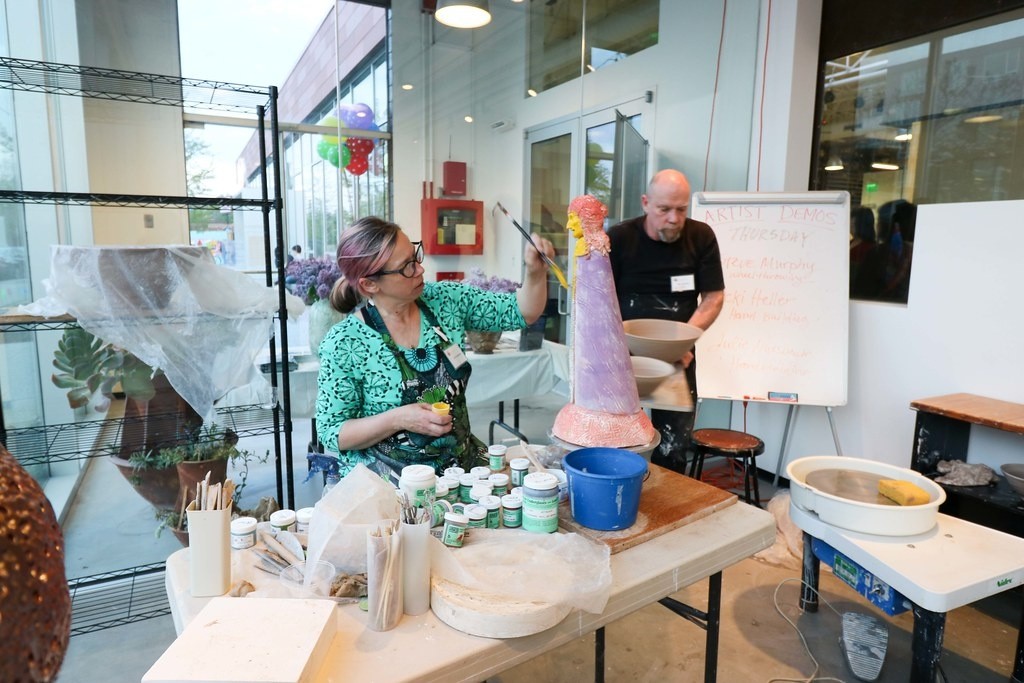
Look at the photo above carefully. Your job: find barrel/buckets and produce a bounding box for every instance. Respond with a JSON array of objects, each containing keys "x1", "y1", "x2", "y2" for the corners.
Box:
[{"x1": 562, "y1": 447, "x2": 651, "y2": 531}]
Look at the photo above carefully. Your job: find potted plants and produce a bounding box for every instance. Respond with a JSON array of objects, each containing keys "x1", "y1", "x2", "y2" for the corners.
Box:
[{"x1": 105, "y1": 419, "x2": 269, "y2": 548}]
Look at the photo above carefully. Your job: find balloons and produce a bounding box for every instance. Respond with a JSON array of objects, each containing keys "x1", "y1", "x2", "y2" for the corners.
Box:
[{"x1": 316, "y1": 102, "x2": 381, "y2": 177}]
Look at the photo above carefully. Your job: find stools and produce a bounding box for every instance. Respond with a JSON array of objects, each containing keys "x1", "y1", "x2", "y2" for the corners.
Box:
[{"x1": 689, "y1": 428, "x2": 765, "y2": 508}]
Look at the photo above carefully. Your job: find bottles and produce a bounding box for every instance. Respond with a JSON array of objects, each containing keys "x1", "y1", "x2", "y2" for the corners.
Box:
[{"x1": 230, "y1": 445, "x2": 559, "y2": 548}]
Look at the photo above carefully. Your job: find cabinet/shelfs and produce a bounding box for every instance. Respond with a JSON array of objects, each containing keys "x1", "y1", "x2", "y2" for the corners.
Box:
[
  {"x1": 0, "y1": 56, "x2": 295, "y2": 637},
  {"x1": 909, "y1": 393, "x2": 1024, "y2": 539}
]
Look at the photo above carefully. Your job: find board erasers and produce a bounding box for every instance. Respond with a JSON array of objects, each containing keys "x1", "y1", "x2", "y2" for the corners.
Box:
[{"x1": 768, "y1": 392, "x2": 798, "y2": 404}]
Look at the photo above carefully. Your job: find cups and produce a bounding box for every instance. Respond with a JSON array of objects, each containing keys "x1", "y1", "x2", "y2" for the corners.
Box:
[
  {"x1": 368, "y1": 518, "x2": 403, "y2": 631},
  {"x1": 432, "y1": 402, "x2": 450, "y2": 417},
  {"x1": 279, "y1": 560, "x2": 336, "y2": 599},
  {"x1": 185, "y1": 498, "x2": 233, "y2": 596},
  {"x1": 400, "y1": 509, "x2": 431, "y2": 615}
]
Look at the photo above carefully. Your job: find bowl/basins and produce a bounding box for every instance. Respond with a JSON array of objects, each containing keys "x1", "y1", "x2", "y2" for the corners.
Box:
[
  {"x1": 629, "y1": 355, "x2": 676, "y2": 396},
  {"x1": 536, "y1": 469, "x2": 568, "y2": 503},
  {"x1": 622, "y1": 319, "x2": 703, "y2": 363},
  {"x1": 1000, "y1": 463, "x2": 1024, "y2": 502}
]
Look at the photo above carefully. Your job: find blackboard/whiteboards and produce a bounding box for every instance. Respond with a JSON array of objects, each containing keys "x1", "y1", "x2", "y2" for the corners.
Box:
[{"x1": 689, "y1": 191, "x2": 851, "y2": 408}]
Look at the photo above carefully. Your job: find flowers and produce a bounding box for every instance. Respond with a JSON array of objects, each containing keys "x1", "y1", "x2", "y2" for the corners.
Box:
[
  {"x1": 460, "y1": 267, "x2": 522, "y2": 293},
  {"x1": 274, "y1": 256, "x2": 342, "y2": 307}
]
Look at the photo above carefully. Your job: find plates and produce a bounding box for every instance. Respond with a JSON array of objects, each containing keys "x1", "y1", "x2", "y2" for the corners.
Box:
[{"x1": 505, "y1": 444, "x2": 547, "y2": 464}]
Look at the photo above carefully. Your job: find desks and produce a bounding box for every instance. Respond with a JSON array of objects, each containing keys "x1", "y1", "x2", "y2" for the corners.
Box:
[
  {"x1": 165, "y1": 449, "x2": 776, "y2": 683},
  {"x1": 254, "y1": 344, "x2": 560, "y2": 486},
  {"x1": 789, "y1": 500, "x2": 1024, "y2": 683}
]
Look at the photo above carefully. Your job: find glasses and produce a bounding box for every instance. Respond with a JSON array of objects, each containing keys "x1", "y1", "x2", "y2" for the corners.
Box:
[{"x1": 365, "y1": 240, "x2": 424, "y2": 278}]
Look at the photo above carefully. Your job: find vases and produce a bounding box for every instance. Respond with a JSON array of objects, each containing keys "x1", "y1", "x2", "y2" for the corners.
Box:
[
  {"x1": 465, "y1": 331, "x2": 502, "y2": 354},
  {"x1": 308, "y1": 298, "x2": 343, "y2": 355}
]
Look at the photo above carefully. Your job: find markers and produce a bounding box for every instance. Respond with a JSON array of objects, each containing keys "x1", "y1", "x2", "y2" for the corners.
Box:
[
  {"x1": 743, "y1": 395, "x2": 765, "y2": 400},
  {"x1": 716, "y1": 395, "x2": 731, "y2": 398}
]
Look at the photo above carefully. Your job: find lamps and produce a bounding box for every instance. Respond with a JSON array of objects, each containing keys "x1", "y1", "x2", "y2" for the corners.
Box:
[
  {"x1": 824, "y1": 155, "x2": 844, "y2": 170},
  {"x1": 435, "y1": 0, "x2": 491, "y2": 29}
]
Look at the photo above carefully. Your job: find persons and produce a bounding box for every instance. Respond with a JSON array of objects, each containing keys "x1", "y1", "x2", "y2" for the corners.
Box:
[
  {"x1": 291, "y1": 245, "x2": 305, "y2": 263},
  {"x1": 314, "y1": 214, "x2": 555, "y2": 489},
  {"x1": 604, "y1": 167, "x2": 726, "y2": 477},
  {"x1": 849, "y1": 199, "x2": 919, "y2": 305}
]
